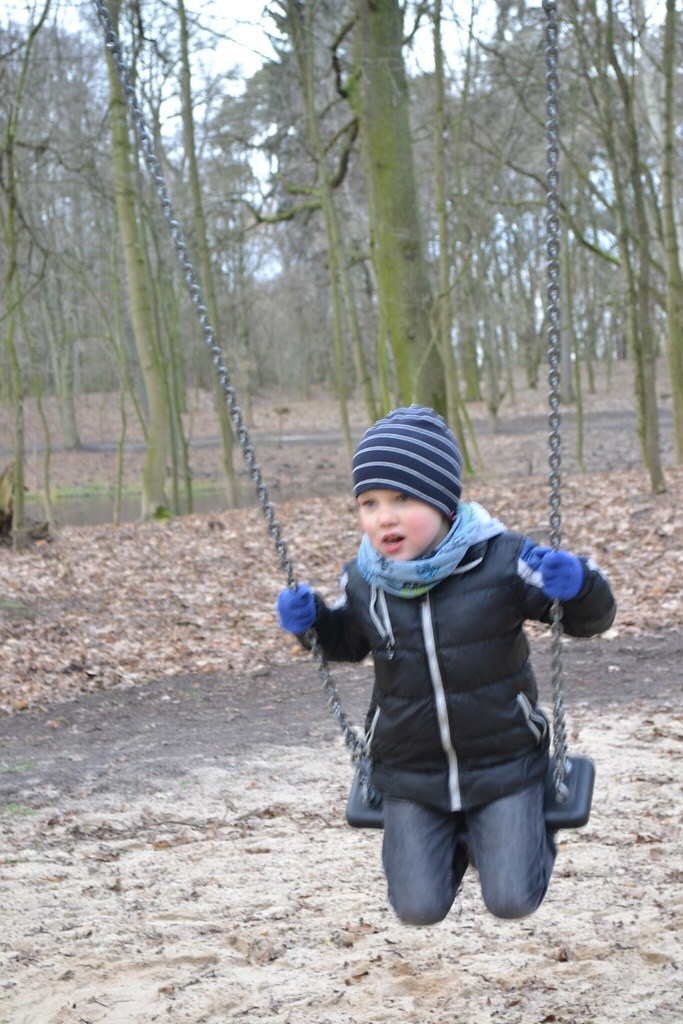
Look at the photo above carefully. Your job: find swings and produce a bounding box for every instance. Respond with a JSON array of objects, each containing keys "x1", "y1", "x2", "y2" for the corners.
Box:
[{"x1": 97, "y1": 1, "x2": 595, "y2": 830}]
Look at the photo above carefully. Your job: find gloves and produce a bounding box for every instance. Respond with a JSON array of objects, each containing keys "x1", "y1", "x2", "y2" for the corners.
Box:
[
  {"x1": 541, "y1": 551, "x2": 583, "y2": 600},
  {"x1": 278, "y1": 581, "x2": 316, "y2": 635}
]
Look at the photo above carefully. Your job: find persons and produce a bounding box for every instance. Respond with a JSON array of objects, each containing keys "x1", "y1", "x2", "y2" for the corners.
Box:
[{"x1": 276, "y1": 403, "x2": 616, "y2": 927}]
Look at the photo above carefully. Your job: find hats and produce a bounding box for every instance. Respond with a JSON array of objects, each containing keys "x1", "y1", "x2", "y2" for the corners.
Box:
[{"x1": 351, "y1": 404, "x2": 464, "y2": 521}]
